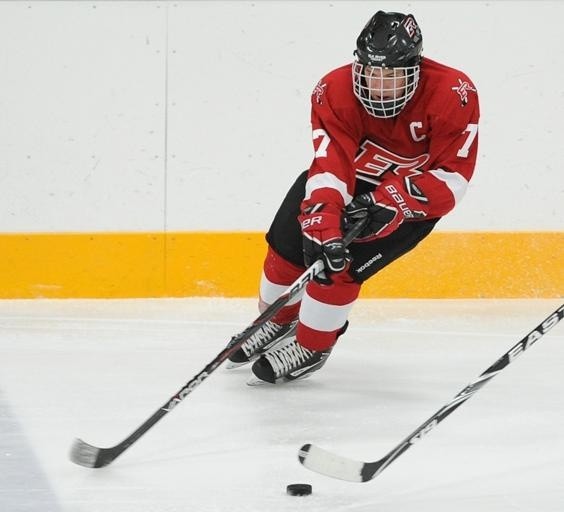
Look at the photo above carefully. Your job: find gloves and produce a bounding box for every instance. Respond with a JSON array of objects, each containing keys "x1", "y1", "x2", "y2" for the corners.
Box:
[{"x1": 297, "y1": 177, "x2": 428, "y2": 286}]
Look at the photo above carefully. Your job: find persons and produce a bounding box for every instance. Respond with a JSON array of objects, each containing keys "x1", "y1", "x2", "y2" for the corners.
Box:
[{"x1": 226, "y1": 11, "x2": 480, "y2": 385}]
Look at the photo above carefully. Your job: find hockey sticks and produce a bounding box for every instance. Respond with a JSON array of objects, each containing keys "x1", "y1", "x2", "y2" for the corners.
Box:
[
  {"x1": 66, "y1": 217, "x2": 372, "y2": 468},
  {"x1": 297, "y1": 304, "x2": 563, "y2": 482}
]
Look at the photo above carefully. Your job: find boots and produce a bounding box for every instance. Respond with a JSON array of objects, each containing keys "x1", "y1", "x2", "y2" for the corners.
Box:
[{"x1": 224, "y1": 319, "x2": 349, "y2": 384}]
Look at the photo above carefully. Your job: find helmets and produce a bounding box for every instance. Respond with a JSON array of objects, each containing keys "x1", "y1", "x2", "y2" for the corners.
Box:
[{"x1": 357, "y1": 10, "x2": 423, "y2": 71}]
